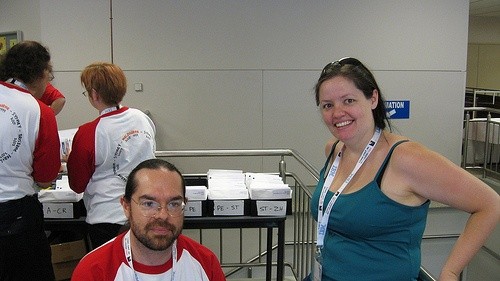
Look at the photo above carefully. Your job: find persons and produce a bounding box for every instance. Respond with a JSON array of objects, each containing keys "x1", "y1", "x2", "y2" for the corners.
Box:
[
  {"x1": 71, "y1": 159, "x2": 226, "y2": 281},
  {"x1": 302, "y1": 57, "x2": 500, "y2": 281},
  {"x1": 0, "y1": 41, "x2": 65, "y2": 281},
  {"x1": 62, "y1": 63, "x2": 157, "y2": 252}
]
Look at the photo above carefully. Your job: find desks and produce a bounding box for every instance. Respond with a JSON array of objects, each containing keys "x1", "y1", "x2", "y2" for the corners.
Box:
[{"x1": 44, "y1": 215, "x2": 287, "y2": 281}]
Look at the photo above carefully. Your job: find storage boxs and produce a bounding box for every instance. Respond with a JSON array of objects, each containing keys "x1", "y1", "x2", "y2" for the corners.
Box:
[
  {"x1": 49, "y1": 233, "x2": 87, "y2": 281},
  {"x1": 40, "y1": 179, "x2": 293, "y2": 220}
]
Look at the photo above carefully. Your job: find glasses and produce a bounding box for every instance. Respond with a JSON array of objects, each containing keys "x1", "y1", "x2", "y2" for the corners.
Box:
[
  {"x1": 129, "y1": 199, "x2": 187, "y2": 218},
  {"x1": 48, "y1": 71, "x2": 56, "y2": 81},
  {"x1": 81, "y1": 89, "x2": 90, "y2": 96},
  {"x1": 320, "y1": 56, "x2": 363, "y2": 76}
]
